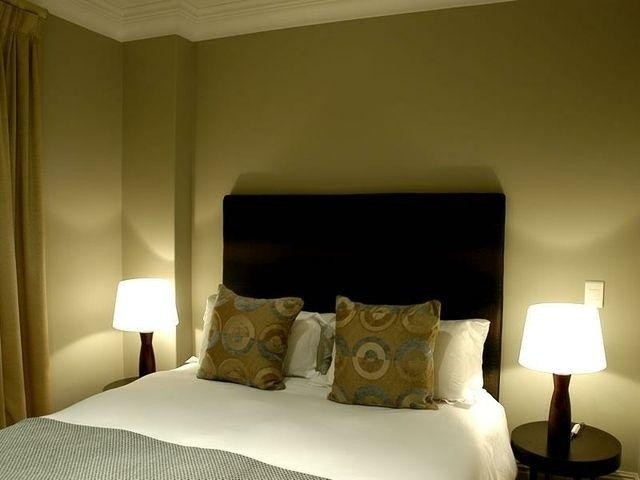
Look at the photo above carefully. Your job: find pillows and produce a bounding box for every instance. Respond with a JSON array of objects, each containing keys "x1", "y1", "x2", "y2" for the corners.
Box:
[{"x1": 195, "y1": 284, "x2": 491, "y2": 410}]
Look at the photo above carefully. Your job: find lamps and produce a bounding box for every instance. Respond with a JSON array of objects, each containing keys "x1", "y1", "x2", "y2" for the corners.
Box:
[
  {"x1": 112, "y1": 278, "x2": 181, "y2": 378},
  {"x1": 519, "y1": 302, "x2": 607, "y2": 474}
]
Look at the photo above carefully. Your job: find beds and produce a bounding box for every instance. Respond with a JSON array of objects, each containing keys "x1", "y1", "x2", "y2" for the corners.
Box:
[{"x1": 0, "y1": 193, "x2": 516, "y2": 480}]
